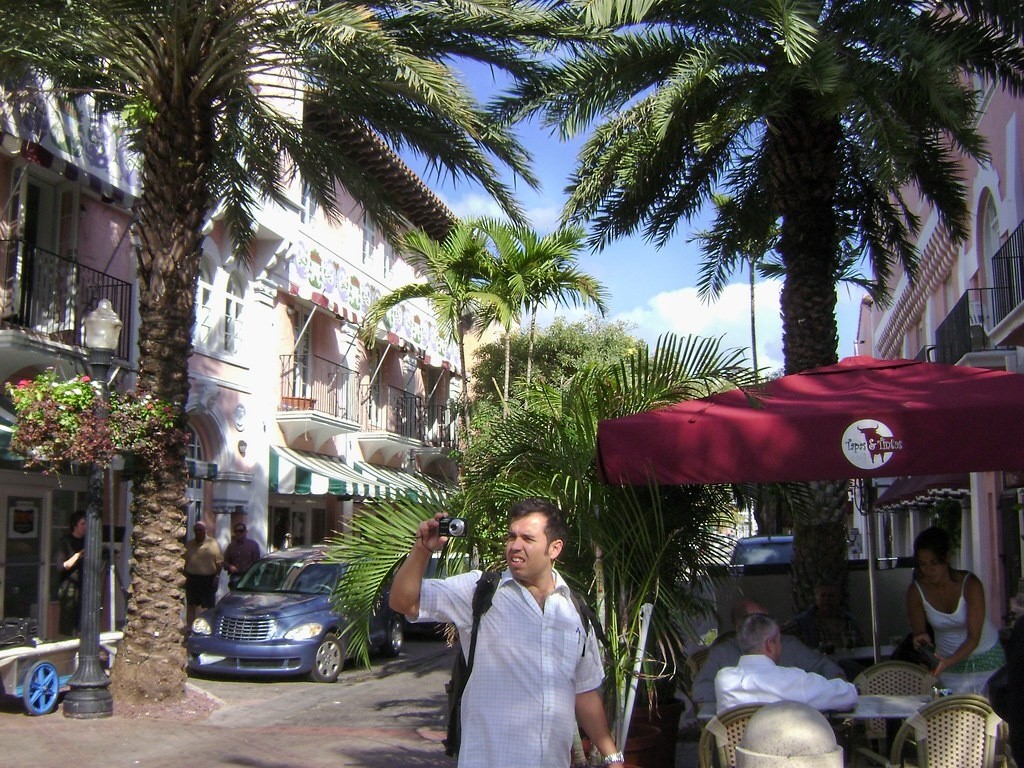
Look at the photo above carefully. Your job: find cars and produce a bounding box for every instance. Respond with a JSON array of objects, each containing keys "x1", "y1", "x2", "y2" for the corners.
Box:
[
  {"x1": 187, "y1": 548, "x2": 403, "y2": 683},
  {"x1": 730, "y1": 537, "x2": 795, "y2": 564}
]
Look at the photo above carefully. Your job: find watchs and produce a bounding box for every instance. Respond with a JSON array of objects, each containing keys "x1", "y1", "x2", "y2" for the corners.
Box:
[{"x1": 601, "y1": 750, "x2": 624, "y2": 767}]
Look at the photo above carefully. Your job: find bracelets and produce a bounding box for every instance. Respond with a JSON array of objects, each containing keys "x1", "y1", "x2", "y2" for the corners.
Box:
[{"x1": 216, "y1": 575, "x2": 221, "y2": 579}]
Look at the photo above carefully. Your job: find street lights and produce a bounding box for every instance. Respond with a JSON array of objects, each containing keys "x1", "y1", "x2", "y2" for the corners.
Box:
[{"x1": 64, "y1": 300, "x2": 123, "y2": 721}]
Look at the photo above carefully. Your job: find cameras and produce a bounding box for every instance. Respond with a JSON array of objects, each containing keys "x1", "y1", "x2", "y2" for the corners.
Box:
[{"x1": 439, "y1": 517, "x2": 467, "y2": 536}]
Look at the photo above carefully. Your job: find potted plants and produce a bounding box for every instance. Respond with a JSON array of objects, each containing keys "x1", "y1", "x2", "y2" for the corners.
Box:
[{"x1": 305, "y1": 332, "x2": 775, "y2": 768}]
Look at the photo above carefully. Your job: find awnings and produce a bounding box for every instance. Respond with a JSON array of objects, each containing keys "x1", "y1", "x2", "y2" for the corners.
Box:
[
  {"x1": 274, "y1": 281, "x2": 367, "y2": 392},
  {"x1": 268, "y1": 443, "x2": 465, "y2": 508},
  {"x1": 360, "y1": 323, "x2": 426, "y2": 405},
  {"x1": 872, "y1": 473, "x2": 970, "y2": 513},
  {"x1": 418, "y1": 346, "x2": 472, "y2": 429},
  {"x1": 0, "y1": 394, "x2": 218, "y2": 481},
  {"x1": 0, "y1": 64, "x2": 145, "y2": 339}
]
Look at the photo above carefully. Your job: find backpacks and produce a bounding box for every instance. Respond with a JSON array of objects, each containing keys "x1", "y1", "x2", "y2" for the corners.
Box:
[{"x1": 441, "y1": 569, "x2": 590, "y2": 757}]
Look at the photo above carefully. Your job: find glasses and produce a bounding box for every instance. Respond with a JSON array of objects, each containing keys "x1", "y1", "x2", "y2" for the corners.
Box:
[{"x1": 233, "y1": 529, "x2": 244, "y2": 534}]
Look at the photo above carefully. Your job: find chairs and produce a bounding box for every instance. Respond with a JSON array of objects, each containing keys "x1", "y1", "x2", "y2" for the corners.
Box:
[
  {"x1": 847, "y1": 693, "x2": 1010, "y2": 768},
  {"x1": 697, "y1": 701, "x2": 770, "y2": 768},
  {"x1": 685, "y1": 649, "x2": 709, "y2": 674},
  {"x1": 850, "y1": 660, "x2": 949, "y2": 758}
]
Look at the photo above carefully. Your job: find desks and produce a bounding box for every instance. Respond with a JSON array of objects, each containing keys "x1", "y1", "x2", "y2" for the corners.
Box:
[
  {"x1": 284, "y1": 396, "x2": 317, "y2": 412},
  {"x1": 696, "y1": 695, "x2": 934, "y2": 718},
  {"x1": 826, "y1": 645, "x2": 897, "y2": 660}
]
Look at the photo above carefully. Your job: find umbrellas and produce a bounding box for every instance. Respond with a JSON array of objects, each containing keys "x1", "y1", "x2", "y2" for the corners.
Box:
[{"x1": 590, "y1": 354, "x2": 1024, "y2": 664}]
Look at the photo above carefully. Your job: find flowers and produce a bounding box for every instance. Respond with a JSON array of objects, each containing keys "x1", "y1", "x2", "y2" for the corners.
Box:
[
  {"x1": 4, "y1": 366, "x2": 114, "y2": 489},
  {"x1": 109, "y1": 389, "x2": 193, "y2": 475}
]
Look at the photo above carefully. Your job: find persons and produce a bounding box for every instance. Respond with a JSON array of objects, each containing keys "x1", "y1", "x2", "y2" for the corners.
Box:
[
  {"x1": 690, "y1": 597, "x2": 860, "y2": 718},
  {"x1": 281, "y1": 533, "x2": 292, "y2": 548},
  {"x1": 53, "y1": 511, "x2": 86, "y2": 642},
  {"x1": 224, "y1": 523, "x2": 261, "y2": 592},
  {"x1": 181, "y1": 520, "x2": 224, "y2": 632},
  {"x1": 906, "y1": 526, "x2": 1007, "y2": 701},
  {"x1": 388, "y1": 499, "x2": 627, "y2": 768}
]
